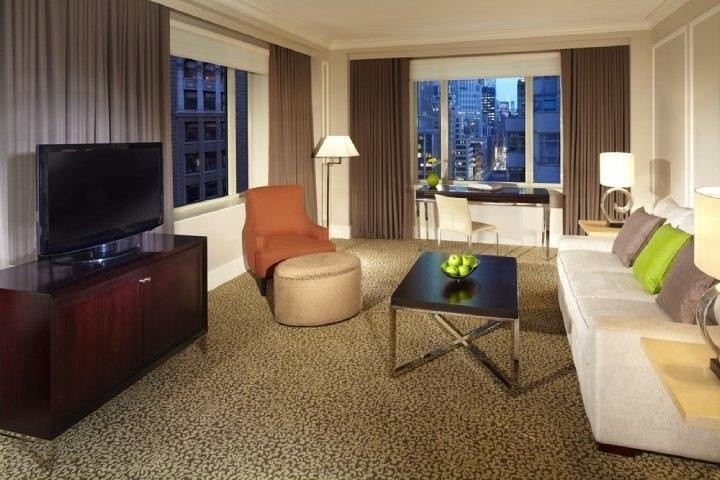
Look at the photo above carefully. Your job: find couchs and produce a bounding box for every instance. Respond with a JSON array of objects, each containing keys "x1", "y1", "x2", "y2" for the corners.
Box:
[{"x1": 552, "y1": 195, "x2": 719, "y2": 465}]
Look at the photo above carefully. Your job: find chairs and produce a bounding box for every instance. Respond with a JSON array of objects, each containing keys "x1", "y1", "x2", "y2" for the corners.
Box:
[
  {"x1": 432, "y1": 191, "x2": 505, "y2": 259},
  {"x1": 241, "y1": 181, "x2": 337, "y2": 298}
]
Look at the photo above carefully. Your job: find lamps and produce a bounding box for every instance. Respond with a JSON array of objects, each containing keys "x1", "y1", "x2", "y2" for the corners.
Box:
[
  {"x1": 308, "y1": 132, "x2": 361, "y2": 229},
  {"x1": 596, "y1": 150, "x2": 641, "y2": 230},
  {"x1": 688, "y1": 185, "x2": 719, "y2": 380}
]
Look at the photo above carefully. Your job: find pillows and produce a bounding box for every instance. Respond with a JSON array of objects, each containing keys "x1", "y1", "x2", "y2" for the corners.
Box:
[
  {"x1": 652, "y1": 239, "x2": 717, "y2": 326},
  {"x1": 610, "y1": 205, "x2": 667, "y2": 268},
  {"x1": 631, "y1": 222, "x2": 694, "y2": 296}
]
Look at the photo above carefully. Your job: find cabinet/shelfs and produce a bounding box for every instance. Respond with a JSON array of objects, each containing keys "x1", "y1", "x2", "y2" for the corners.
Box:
[{"x1": 1, "y1": 231, "x2": 211, "y2": 441}]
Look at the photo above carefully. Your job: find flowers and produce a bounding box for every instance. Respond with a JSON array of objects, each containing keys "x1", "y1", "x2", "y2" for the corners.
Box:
[{"x1": 424, "y1": 157, "x2": 439, "y2": 172}]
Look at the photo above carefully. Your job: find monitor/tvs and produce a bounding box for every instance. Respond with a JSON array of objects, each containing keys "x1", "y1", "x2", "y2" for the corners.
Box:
[{"x1": 35, "y1": 141, "x2": 164, "y2": 265}]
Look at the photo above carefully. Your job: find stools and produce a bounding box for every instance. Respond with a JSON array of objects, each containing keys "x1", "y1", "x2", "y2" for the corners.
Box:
[{"x1": 270, "y1": 250, "x2": 366, "y2": 330}]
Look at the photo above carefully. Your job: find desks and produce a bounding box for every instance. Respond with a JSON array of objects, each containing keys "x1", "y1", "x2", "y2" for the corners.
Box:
[
  {"x1": 633, "y1": 334, "x2": 719, "y2": 433},
  {"x1": 412, "y1": 182, "x2": 552, "y2": 263},
  {"x1": 575, "y1": 218, "x2": 621, "y2": 237}
]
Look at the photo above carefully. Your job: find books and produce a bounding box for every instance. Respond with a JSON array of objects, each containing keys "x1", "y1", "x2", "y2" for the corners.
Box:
[{"x1": 469, "y1": 182, "x2": 502, "y2": 191}]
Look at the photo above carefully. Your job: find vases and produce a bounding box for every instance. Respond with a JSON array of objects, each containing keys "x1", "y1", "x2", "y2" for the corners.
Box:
[{"x1": 425, "y1": 171, "x2": 439, "y2": 187}]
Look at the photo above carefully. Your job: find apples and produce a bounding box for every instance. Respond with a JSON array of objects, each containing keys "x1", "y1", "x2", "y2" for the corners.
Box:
[{"x1": 441, "y1": 255, "x2": 475, "y2": 277}]
